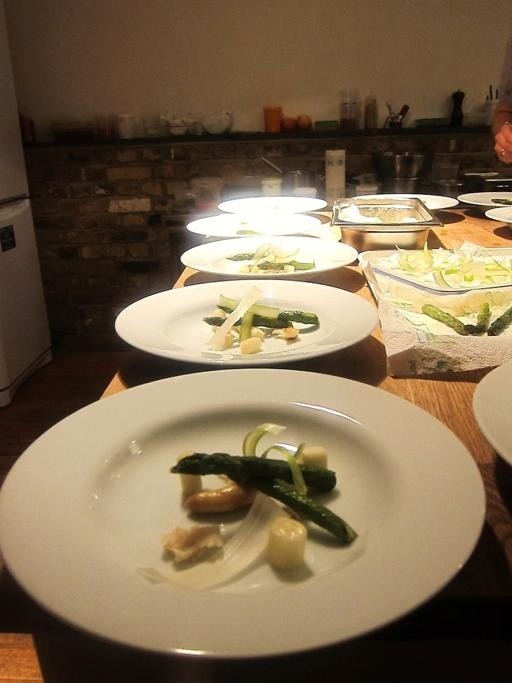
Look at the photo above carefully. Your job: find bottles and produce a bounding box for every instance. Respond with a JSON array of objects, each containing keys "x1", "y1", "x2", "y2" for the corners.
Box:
[
  {"x1": 365, "y1": 95, "x2": 378, "y2": 130},
  {"x1": 340, "y1": 90, "x2": 359, "y2": 129}
]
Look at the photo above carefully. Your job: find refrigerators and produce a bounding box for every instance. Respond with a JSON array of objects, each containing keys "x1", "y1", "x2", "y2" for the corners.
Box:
[{"x1": 0, "y1": 22, "x2": 54, "y2": 408}]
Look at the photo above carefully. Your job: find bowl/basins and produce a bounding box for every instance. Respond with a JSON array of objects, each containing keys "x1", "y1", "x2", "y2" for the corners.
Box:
[
  {"x1": 341, "y1": 227, "x2": 429, "y2": 251},
  {"x1": 370, "y1": 151, "x2": 425, "y2": 179}
]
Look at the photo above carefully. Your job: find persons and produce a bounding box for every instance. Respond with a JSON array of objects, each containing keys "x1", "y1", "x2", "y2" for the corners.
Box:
[{"x1": 490, "y1": 33, "x2": 512, "y2": 168}]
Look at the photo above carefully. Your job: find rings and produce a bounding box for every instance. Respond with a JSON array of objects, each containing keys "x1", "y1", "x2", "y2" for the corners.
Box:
[{"x1": 500, "y1": 149, "x2": 505, "y2": 156}]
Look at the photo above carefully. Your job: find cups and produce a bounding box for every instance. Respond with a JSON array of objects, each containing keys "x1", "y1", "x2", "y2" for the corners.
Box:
[
  {"x1": 143, "y1": 113, "x2": 162, "y2": 138},
  {"x1": 264, "y1": 105, "x2": 281, "y2": 133},
  {"x1": 95, "y1": 111, "x2": 114, "y2": 138}
]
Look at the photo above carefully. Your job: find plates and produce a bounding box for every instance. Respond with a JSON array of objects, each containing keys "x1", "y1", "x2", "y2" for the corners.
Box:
[
  {"x1": 217, "y1": 196, "x2": 328, "y2": 214},
  {"x1": 355, "y1": 194, "x2": 458, "y2": 211},
  {"x1": 483, "y1": 208, "x2": 512, "y2": 225},
  {"x1": 369, "y1": 256, "x2": 512, "y2": 293},
  {"x1": 457, "y1": 191, "x2": 512, "y2": 211},
  {"x1": 472, "y1": 357, "x2": 510, "y2": 467},
  {"x1": 0, "y1": 369, "x2": 486, "y2": 662},
  {"x1": 181, "y1": 235, "x2": 361, "y2": 278},
  {"x1": 114, "y1": 278, "x2": 379, "y2": 369},
  {"x1": 187, "y1": 215, "x2": 323, "y2": 237},
  {"x1": 331, "y1": 196, "x2": 443, "y2": 231}
]
